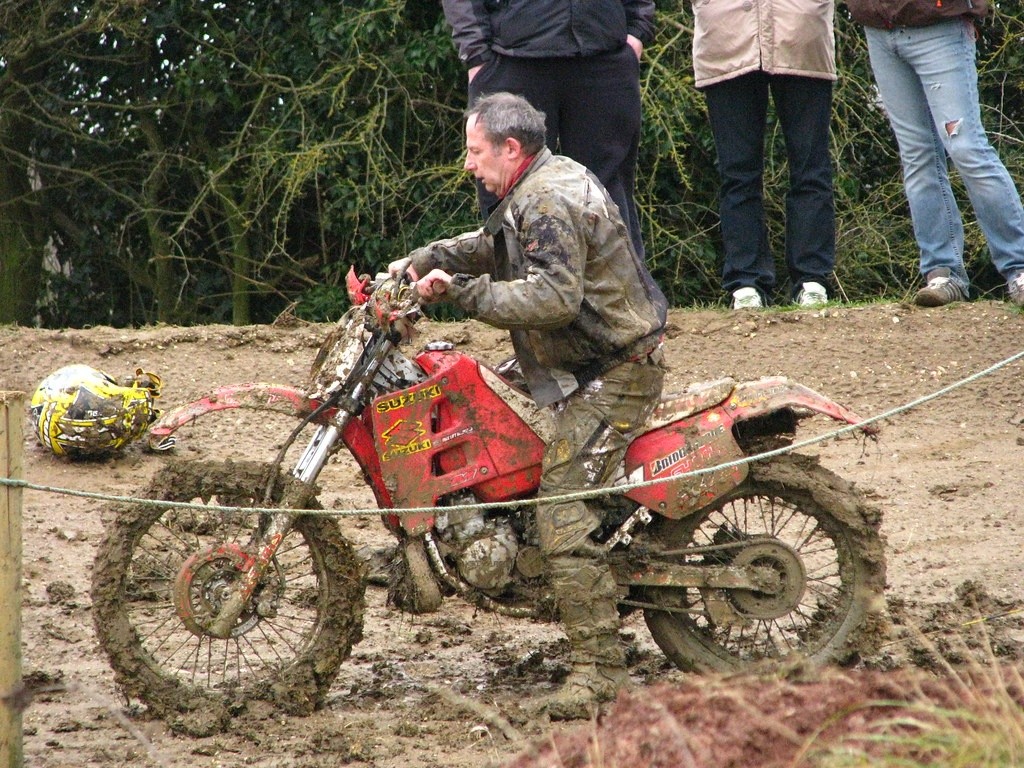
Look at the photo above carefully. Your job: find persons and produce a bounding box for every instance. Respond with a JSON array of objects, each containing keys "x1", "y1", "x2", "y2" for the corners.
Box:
[
  {"x1": 845, "y1": 0, "x2": 1024, "y2": 307},
  {"x1": 438, "y1": 0, "x2": 657, "y2": 264},
  {"x1": 388, "y1": 91, "x2": 670, "y2": 722},
  {"x1": 689, "y1": 0, "x2": 835, "y2": 309}
]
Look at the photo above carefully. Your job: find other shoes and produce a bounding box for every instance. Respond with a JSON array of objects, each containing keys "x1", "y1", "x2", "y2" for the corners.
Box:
[
  {"x1": 796, "y1": 281, "x2": 828, "y2": 306},
  {"x1": 535, "y1": 666, "x2": 635, "y2": 720},
  {"x1": 732, "y1": 287, "x2": 762, "y2": 310},
  {"x1": 914, "y1": 267, "x2": 967, "y2": 305},
  {"x1": 1009, "y1": 269, "x2": 1024, "y2": 306}
]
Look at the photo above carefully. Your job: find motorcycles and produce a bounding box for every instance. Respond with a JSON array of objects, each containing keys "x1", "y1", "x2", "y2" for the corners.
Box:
[{"x1": 87, "y1": 260, "x2": 892, "y2": 721}]
